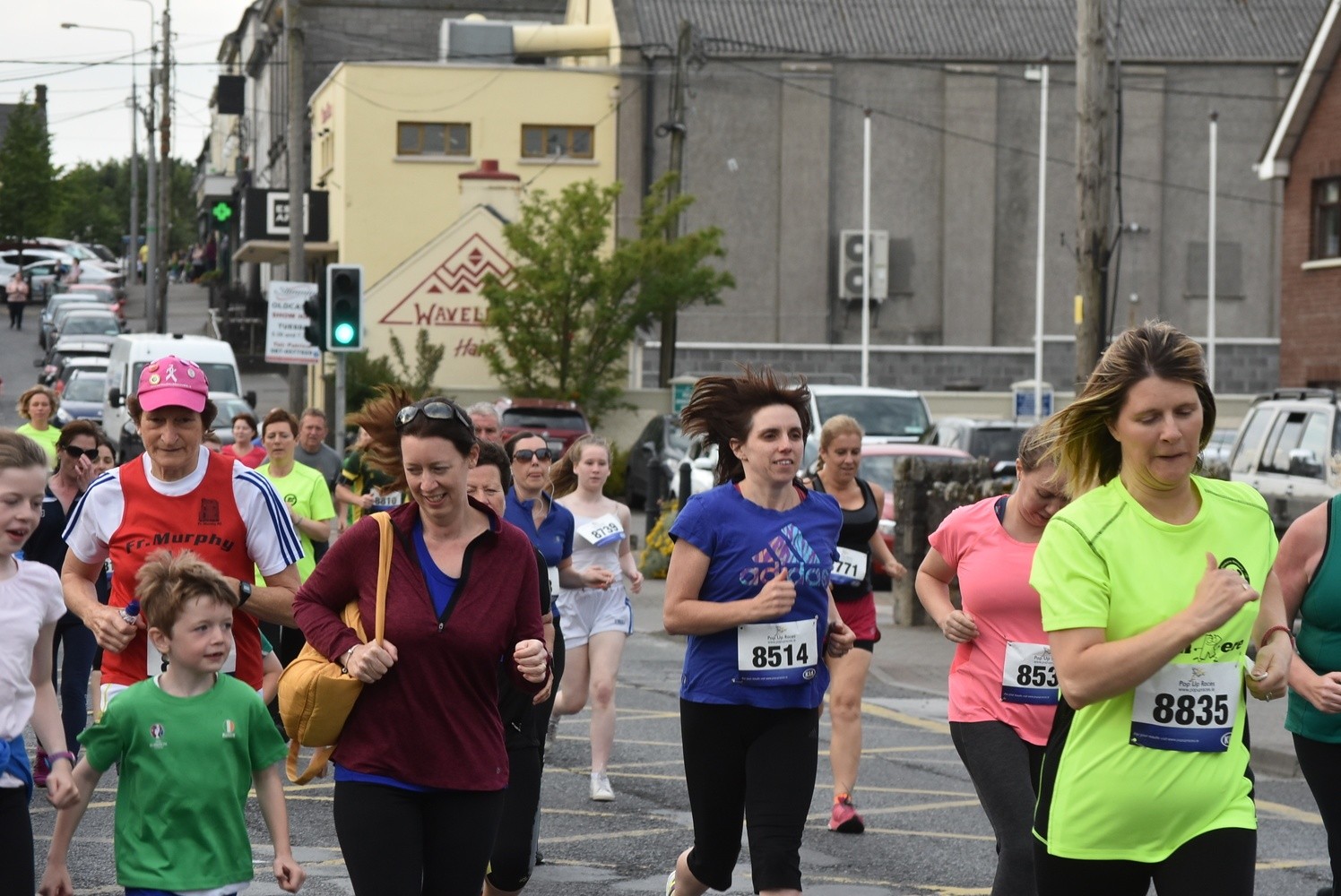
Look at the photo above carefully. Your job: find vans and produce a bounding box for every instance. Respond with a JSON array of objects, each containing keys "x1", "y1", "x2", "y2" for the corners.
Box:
[{"x1": 104, "y1": 331, "x2": 259, "y2": 461}]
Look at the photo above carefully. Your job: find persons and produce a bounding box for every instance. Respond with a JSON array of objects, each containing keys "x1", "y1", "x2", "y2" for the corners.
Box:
[
  {"x1": 663, "y1": 378, "x2": 856, "y2": 896},
  {"x1": 1028, "y1": 322, "x2": 1293, "y2": 896},
  {"x1": 803, "y1": 415, "x2": 907, "y2": 832},
  {"x1": 916, "y1": 427, "x2": 1081, "y2": 896},
  {"x1": 5, "y1": 272, "x2": 28, "y2": 329},
  {"x1": 543, "y1": 435, "x2": 644, "y2": 801},
  {"x1": 0, "y1": 355, "x2": 613, "y2": 896},
  {"x1": 52, "y1": 242, "x2": 206, "y2": 292},
  {"x1": 1274, "y1": 449, "x2": 1341, "y2": 896}
]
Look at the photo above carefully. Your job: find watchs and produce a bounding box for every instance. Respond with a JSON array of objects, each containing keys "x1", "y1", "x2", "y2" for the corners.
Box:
[
  {"x1": 236, "y1": 580, "x2": 251, "y2": 608},
  {"x1": 45, "y1": 752, "x2": 75, "y2": 769}
]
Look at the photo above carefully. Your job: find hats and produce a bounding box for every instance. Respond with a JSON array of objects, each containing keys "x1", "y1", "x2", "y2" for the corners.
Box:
[{"x1": 136, "y1": 354, "x2": 208, "y2": 413}]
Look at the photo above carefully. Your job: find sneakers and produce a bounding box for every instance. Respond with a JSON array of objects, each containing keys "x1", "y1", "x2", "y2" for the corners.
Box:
[
  {"x1": 590, "y1": 763, "x2": 615, "y2": 801},
  {"x1": 544, "y1": 717, "x2": 561, "y2": 751},
  {"x1": 828, "y1": 793, "x2": 865, "y2": 834}
]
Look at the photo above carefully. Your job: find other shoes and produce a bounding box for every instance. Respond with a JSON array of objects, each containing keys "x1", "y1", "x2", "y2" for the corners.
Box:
[
  {"x1": 34, "y1": 745, "x2": 50, "y2": 788},
  {"x1": 665, "y1": 870, "x2": 675, "y2": 896}
]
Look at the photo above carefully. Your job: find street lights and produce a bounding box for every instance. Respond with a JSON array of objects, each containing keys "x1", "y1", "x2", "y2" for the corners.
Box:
[
  {"x1": 1023, "y1": 64, "x2": 1048, "y2": 427},
  {"x1": 60, "y1": 22, "x2": 139, "y2": 296}
]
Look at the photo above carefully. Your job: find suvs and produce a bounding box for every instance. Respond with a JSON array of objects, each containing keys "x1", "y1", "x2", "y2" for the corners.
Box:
[{"x1": 489, "y1": 397, "x2": 592, "y2": 472}]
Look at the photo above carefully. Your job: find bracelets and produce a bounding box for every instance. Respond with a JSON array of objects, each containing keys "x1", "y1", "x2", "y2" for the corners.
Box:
[
  {"x1": 294, "y1": 518, "x2": 303, "y2": 525},
  {"x1": 342, "y1": 644, "x2": 359, "y2": 674}
]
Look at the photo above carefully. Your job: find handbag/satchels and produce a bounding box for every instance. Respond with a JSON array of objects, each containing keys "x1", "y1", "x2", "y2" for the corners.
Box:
[{"x1": 275, "y1": 511, "x2": 394, "y2": 786}]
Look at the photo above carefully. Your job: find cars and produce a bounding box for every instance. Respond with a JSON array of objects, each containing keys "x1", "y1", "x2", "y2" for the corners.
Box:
[
  {"x1": 1, "y1": 235, "x2": 130, "y2": 431},
  {"x1": 114, "y1": 389, "x2": 266, "y2": 467},
  {"x1": 619, "y1": 375, "x2": 1341, "y2": 595}
]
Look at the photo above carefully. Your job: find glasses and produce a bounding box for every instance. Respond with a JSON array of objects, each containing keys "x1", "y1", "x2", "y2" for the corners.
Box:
[
  {"x1": 62, "y1": 445, "x2": 99, "y2": 460},
  {"x1": 513, "y1": 448, "x2": 552, "y2": 464},
  {"x1": 394, "y1": 402, "x2": 477, "y2": 442}
]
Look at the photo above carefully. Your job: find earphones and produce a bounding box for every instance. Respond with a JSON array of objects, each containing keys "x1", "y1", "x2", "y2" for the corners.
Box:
[
  {"x1": 548, "y1": 465, "x2": 551, "y2": 474},
  {"x1": 509, "y1": 465, "x2": 513, "y2": 476}
]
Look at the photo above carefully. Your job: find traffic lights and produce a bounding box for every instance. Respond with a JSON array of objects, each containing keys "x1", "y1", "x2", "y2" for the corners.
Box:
[
  {"x1": 324, "y1": 263, "x2": 367, "y2": 352},
  {"x1": 303, "y1": 270, "x2": 325, "y2": 351}
]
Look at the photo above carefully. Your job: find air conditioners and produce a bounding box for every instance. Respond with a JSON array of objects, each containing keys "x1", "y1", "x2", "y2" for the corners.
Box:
[{"x1": 840, "y1": 229, "x2": 889, "y2": 300}]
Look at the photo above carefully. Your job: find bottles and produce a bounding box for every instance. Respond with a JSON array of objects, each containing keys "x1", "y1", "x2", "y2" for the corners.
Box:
[{"x1": 120, "y1": 598, "x2": 141, "y2": 626}]
